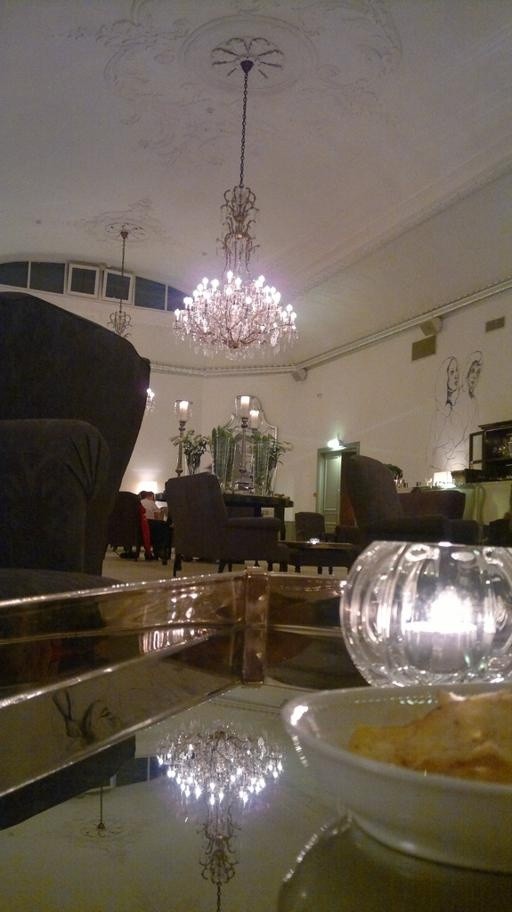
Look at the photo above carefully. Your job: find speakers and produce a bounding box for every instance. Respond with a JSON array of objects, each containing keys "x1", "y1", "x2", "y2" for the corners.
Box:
[
  {"x1": 291, "y1": 369, "x2": 306, "y2": 382},
  {"x1": 419, "y1": 318, "x2": 442, "y2": 336}
]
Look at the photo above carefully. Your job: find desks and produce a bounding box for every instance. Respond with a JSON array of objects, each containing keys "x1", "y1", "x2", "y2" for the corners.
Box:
[{"x1": 1, "y1": 566, "x2": 512, "y2": 912}]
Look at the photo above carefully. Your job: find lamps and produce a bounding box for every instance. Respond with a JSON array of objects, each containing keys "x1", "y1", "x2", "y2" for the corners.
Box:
[
  {"x1": 154, "y1": 725, "x2": 284, "y2": 912},
  {"x1": 103, "y1": 222, "x2": 156, "y2": 416},
  {"x1": 172, "y1": 37, "x2": 296, "y2": 359},
  {"x1": 327, "y1": 434, "x2": 346, "y2": 450}
]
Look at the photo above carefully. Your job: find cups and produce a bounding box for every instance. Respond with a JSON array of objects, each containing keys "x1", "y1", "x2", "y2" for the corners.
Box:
[{"x1": 340, "y1": 541, "x2": 512, "y2": 687}]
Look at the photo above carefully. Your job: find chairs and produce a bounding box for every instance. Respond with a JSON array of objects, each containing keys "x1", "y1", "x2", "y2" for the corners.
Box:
[
  {"x1": 2, "y1": 290, "x2": 152, "y2": 599},
  {"x1": 345, "y1": 455, "x2": 479, "y2": 545},
  {"x1": 114, "y1": 491, "x2": 173, "y2": 561},
  {"x1": 164, "y1": 472, "x2": 282, "y2": 576},
  {"x1": 296, "y1": 512, "x2": 335, "y2": 540}
]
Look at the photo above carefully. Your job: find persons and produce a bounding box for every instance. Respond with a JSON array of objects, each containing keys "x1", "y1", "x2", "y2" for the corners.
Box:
[{"x1": 140, "y1": 491, "x2": 169, "y2": 560}]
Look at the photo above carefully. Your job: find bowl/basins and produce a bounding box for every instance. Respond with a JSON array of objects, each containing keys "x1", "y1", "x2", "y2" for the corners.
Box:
[
  {"x1": 280, "y1": 682, "x2": 512, "y2": 876},
  {"x1": 271, "y1": 820, "x2": 511, "y2": 911}
]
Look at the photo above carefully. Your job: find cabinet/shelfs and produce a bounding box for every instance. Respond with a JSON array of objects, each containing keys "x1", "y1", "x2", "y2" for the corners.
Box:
[{"x1": 469, "y1": 418, "x2": 512, "y2": 480}]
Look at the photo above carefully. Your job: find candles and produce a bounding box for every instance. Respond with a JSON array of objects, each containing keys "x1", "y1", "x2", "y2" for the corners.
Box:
[
  {"x1": 238, "y1": 395, "x2": 249, "y2": 419},
  {"x1": 178, "y1": 400, "x2": 191, "y2": 423},
  {"x1": 250, "y1": 410, "x2": 259, "y2": 429}
]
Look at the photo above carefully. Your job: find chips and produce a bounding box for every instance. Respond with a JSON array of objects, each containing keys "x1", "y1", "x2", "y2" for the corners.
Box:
[{"x1": 345, "y1": 690, "x2": 511, "y2": 784}]
